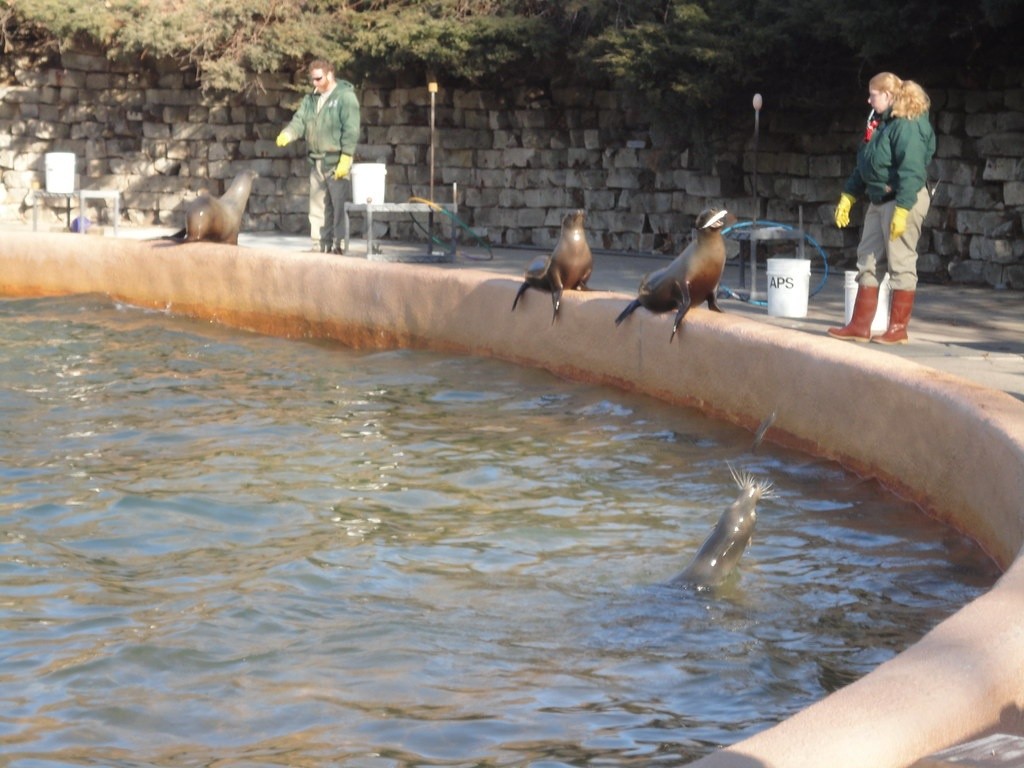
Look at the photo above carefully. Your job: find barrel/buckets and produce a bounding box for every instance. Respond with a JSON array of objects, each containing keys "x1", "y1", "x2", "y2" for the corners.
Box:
[
  {"x1": 766, "y1": 259, "x2": 811, "y2": 318},
  {"x1": 844, "y1": 271, "x2": 890, "y2": 331},
  {"x1": 44, "y1": 152, "x2": 75, "y2": 194},
  {"x1": 351, "y1": 162, "x2": 388, "y2": 204}
]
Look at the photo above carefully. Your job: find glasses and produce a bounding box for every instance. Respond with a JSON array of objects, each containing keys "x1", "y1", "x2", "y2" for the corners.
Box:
[{"x1": 313, "y1": 71, "x2": 328, "y2": 82}]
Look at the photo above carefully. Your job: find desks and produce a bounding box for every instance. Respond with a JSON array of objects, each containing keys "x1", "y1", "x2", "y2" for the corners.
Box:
[
  {"x1": 730, "y1": 227, "x2": 806, "y2": 304},
  {"x1": 31, "y1": 189, "x2": 120, "y2": 235},
  {"x1": 344, "y1": 199, "x2": 458, "y2": 263}
]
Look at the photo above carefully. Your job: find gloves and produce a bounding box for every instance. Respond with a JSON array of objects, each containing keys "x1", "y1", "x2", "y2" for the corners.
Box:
[
  {"x1": 276, "y1": 133, "x2": 289, "y2": 148},
  {"x1": 890, "y1": 205, "x2": 910, "y2": 241},
  {"x1": 834, "y1": 191, "x2": 857, "y2": 228},
  {"x1": 331, "y1": 153, "x2": 354, "y2": 181}
]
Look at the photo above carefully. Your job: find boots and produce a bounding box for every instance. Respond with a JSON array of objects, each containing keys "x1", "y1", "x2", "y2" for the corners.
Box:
[
  {"x1": 871, "y1": 289, "x2": 916, "y2": 344},
  {"x1": 827, "y1": 285, "x2": 881, "y2": 343}
]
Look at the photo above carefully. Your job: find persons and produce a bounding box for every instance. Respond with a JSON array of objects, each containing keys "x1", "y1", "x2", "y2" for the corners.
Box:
[
  {"x1": 827, "y1": 73, "x2": 936, "y2": 344},
  {"x1": 276, "y1": 60, "x2": 360, "y2": 252}
]
